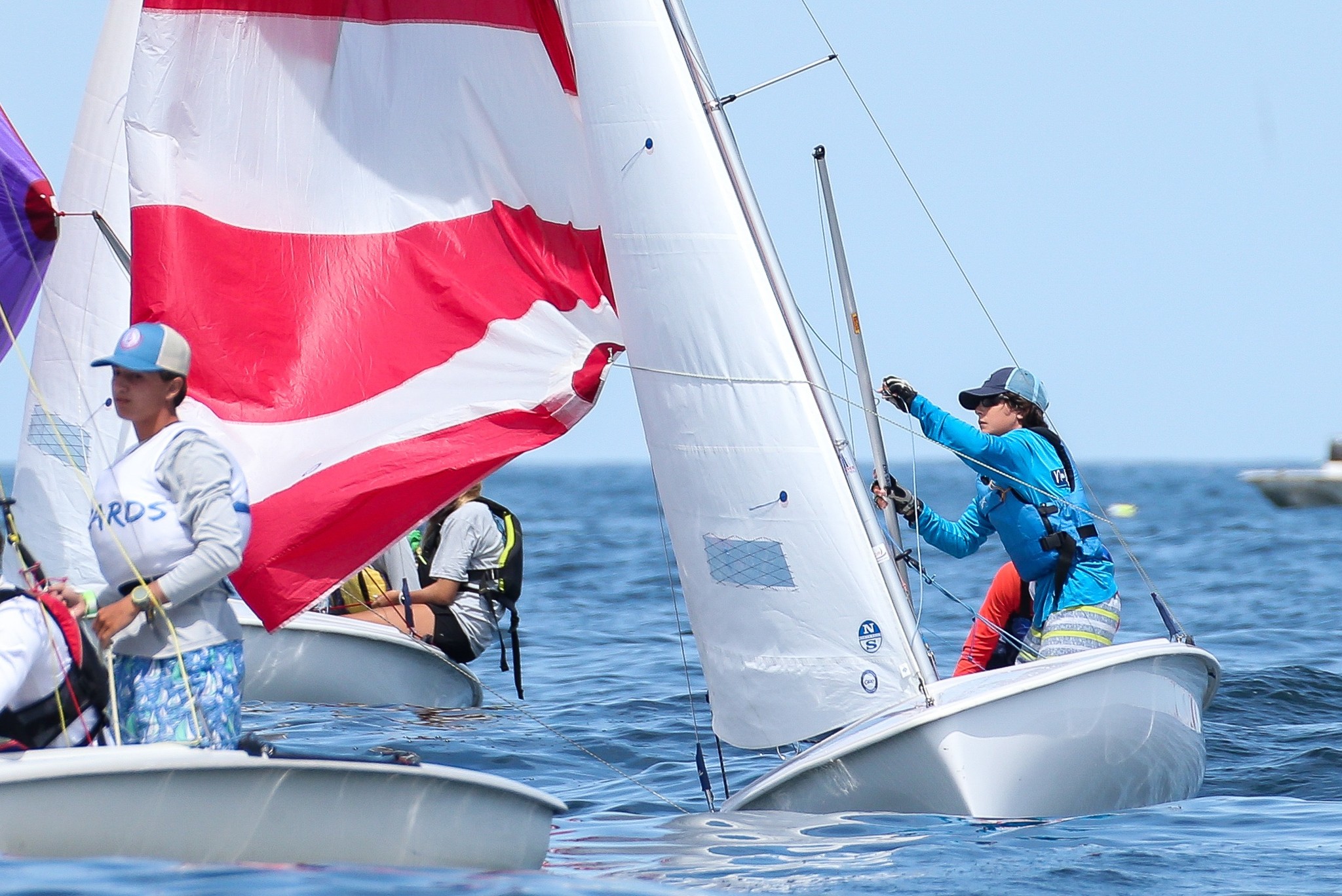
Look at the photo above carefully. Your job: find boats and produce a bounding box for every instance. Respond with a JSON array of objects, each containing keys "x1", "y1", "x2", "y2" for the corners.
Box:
[{"x1": 1233, "y1": 437, "x2": 1342, "y2": 509}]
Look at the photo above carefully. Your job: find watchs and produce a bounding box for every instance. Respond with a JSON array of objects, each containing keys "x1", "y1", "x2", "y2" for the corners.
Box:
[{"x1": 131, "y1": 584, "x2": 151, "y2": 611}]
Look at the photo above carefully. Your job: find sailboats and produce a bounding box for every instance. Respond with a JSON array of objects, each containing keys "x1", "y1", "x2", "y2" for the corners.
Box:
[{"x1": 0, "y1": 1, "x2": 1227, "y2": 867}]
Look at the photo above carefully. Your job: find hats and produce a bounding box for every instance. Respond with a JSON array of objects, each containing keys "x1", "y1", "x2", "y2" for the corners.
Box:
[
  {"x1": 959, "y1": 366, "x2": 1050, "y2": 414},
  {"x1": 91, "y1": 321, "x2": 189, "y2": 375}
]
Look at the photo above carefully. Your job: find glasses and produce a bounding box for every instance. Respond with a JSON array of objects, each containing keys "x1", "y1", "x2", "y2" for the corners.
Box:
[{"x1": 976, "y1": 395, "x2": 1001, "y2": 407}]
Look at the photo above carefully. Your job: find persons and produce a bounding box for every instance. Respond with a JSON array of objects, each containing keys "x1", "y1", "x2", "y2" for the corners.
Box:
[
  {"x1": 871, "y1": 366, "x2": 1122, "y2": 680},
  {"x1": 1, "y1": 537, "x2": 113, "y2": 746},
  {"x1": 41, "y1": 321, "x2": 252, "y2": 750},
  {"x1": 328, "y1": 477, "x2": 511, "y2": 666}
]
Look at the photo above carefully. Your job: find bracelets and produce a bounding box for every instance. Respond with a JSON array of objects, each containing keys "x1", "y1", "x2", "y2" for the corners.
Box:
[{"x1": 84, "y1": 591, "x2": 97, "y2": 614}]
